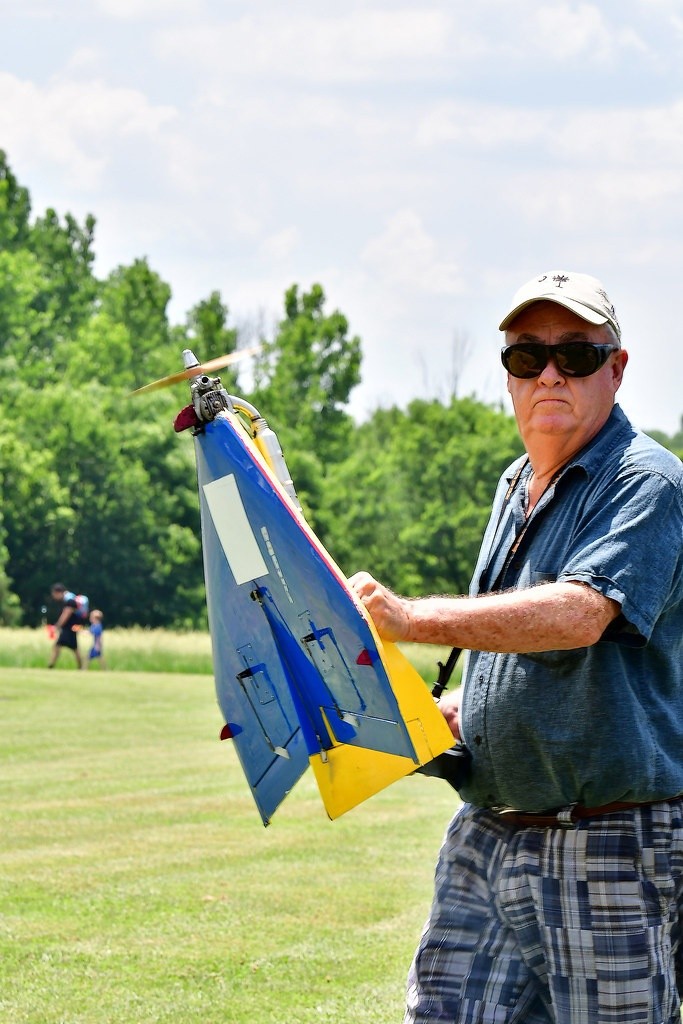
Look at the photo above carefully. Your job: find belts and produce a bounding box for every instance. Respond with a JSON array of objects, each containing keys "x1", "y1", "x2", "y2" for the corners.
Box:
[{"x1": 489, "y1": 799, "x2": 670, "y2": 828}]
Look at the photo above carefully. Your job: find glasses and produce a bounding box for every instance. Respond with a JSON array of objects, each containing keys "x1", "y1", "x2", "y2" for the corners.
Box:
[{"x1": 500, "y1": 341, "x2": 617, "y2": 379}]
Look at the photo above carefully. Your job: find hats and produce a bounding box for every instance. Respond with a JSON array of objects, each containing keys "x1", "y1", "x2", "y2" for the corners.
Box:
[{"x1": 498, "y1": 270, "x2": 620, "y2": 344}]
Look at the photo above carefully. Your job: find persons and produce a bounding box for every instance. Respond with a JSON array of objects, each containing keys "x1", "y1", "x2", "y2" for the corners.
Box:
[
  {"x1": 86, "y1": 610, "x2": 109, "y2": 672},
  {"x1": 347, "y1": 271, "x2": 682, "y2": 1024},
  {"x1": 49, "y1": 582, "x2": 85, "y2": 670}
]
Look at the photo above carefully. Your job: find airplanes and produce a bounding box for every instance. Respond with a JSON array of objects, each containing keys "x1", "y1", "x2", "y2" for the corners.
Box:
[{"x1": 121, "y1": 339, "x2": 457, "y2": 828}]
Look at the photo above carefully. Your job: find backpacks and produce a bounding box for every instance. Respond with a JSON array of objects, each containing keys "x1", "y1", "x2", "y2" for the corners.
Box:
[{"x1": 67, "y1": 593, "x2": 89, "y2": 617}]
